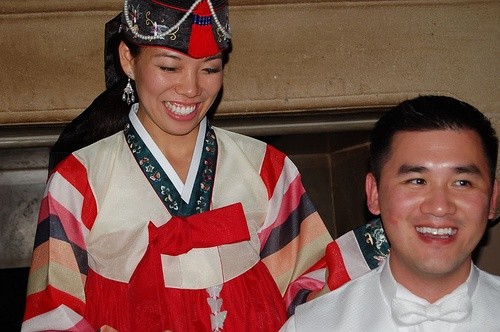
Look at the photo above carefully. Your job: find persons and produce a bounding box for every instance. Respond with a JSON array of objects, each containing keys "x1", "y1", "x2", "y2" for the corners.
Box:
[
  {"x1": 21, "y1": 0, "x2": 390, "y2": 332},
  {"x1": 277, "y1": 95, "x2": 500, "y2": 332}
]
